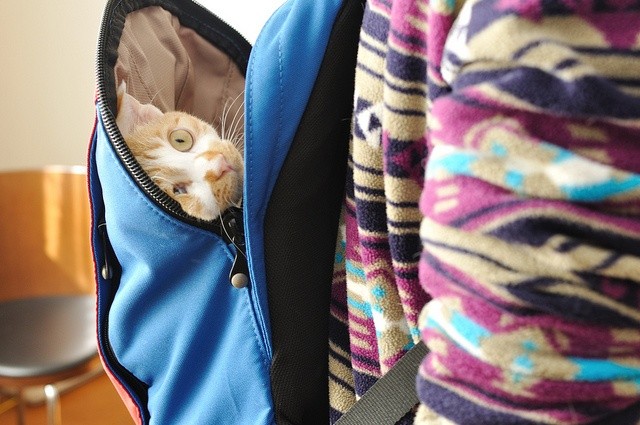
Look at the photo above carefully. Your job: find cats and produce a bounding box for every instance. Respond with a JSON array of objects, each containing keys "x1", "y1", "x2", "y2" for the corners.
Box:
[{"x1": 104, "y1": 78, "x2": 244, "y2": 260}]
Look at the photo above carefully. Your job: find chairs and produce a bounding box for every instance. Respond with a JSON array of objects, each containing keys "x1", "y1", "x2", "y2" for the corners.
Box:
[{"x1": 1, "y1": 171, "x2": 104, "y2": 423}]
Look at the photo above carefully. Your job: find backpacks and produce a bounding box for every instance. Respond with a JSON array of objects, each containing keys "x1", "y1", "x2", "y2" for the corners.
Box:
[{"x1": 87, "y1": 0, "x2": 430, "y2": 425}]
